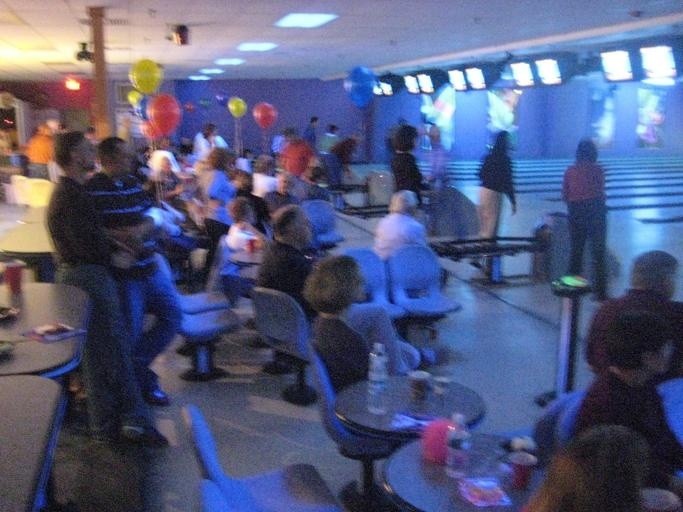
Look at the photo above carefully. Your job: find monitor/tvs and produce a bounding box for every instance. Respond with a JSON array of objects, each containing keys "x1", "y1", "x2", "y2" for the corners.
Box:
[
  {"x1": 534, "y1": 53, "x2": 578, "y2": 85},
  {"x1": 508, "y1": 58, "x2": 536, "y2": 88},
  {"x1": 373, "y1": 73, "x2": 403, "y2": 96},
  {"x1": 597, "y1": 46, "x2": 641, "y2": 83},
  {"x1": 402, "y1": 70, "x2": 420, "y2": 95},
  {"x1": 448, "y1": 66, "x2": 468, "y2": 92},
  {"x1": 464, "y1": 60, "x2": 502, "y2": 90},
  {"x1": 416, "y1": 67, "x2": 448, "y2": 94},
  {"x1": 637, "y1": 43, "x2": 681, "y2": 78}
]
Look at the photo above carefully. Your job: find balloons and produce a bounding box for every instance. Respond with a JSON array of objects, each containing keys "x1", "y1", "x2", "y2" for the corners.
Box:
[
  {"x1": 226, "y1": 94, "x2": 249, "y2": 122},
  {"x1": 184, "y1": 101, "x2": 198, "y2": 113},
  {"x1": 145, "y1": 92, "x2": 181, "y2": 140},
  {"x1": 214, "y1": 88, "x2": 230, "y2": 108},
  {"x1": 251, "y1": 101, "x2": 279, "y2": 131},
  {"x1": 125, "y1": 88, "x2": 142, "y2": 110},
  {"x1": 130, "y1": 59, "x2": 163, "y2": 95},
  {"x1": 199, "y1": 94, "x2": 216, "y2": 111},
  {"x1": 342, "y1": 65, "x2": 381, "y2": 111}
]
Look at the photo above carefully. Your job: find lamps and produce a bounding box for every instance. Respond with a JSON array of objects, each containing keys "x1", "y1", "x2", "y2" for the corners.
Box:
[
  {"x1": 174, "y1": 25, "x2": 189, "y2": 46},
  {"x1": 77, "y1": 43, "x2": 94, "y2": 63}
]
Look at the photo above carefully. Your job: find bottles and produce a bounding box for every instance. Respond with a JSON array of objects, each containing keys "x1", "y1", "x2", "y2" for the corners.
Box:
[
  {"x1": 366, "y1": 342, "x2": 392, "y2": 416},
  {"x1": 442, "y1": 412, "x2": 472, "y2": 479}
]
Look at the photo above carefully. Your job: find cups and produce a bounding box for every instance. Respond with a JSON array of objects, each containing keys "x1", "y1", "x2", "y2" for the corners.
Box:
[
  {"x1": 246, "y1": 238, "x2": 257, "y2": 251},
  {"x1": 509, "y1": 451, "x2": 536, "y2": 488},
  {"x1": 0, "y1": 259, "x2": 26, "y2": 299},
  {"x1": 408, "y1": 370, "x2": 430, "y2": 406},
  {"x1": 434, "y1": 375, "x2": 450, "y2": 395}
]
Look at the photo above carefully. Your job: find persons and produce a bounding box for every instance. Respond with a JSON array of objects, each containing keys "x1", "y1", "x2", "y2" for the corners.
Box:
[
  {"x1": 477, "y1": 131, "x2": 518, "y2": 235},
  {"x1": 389, "y1": 118, "x2": 448, "y2": 199},
  {"x1": 45, "y1": 131, "x2": 181, "y2": 449},
  {"x1": 25, "y1": 123, "x2": 55, "y2": 178},
  {"x1": 559, "y1": 135, "x2": 618, "y2": 302},
  {"x1": 138, "y1": 123, "x2": 281, "y2": 282},
  {"x1": 0, "y1": 131, "x2": 24, "y2": 193},
  {"x1": 582, "y1": 250, "x2": 682, "y2": 386},
  {"x1": 512, "y1": 424, "x2": 656, "y2": 512},
  {"x1": 574, "y1": 307, "x2": 682, "y2": 500},
  {"x1": 0, "y1": 88, "x2": 21, "y2": 147},
  {"x1": 303, "y1": 252, "x2": 372, "y2": 394},
  {"x1": 262, "y1": 205, "x2": 415, "y2": 377},
  {"x1": 272, "y1": 116, "x2": 361, "y2": 191},
  {"x1": 374, "y1": 191, "x2": 448, "y2": 284}
]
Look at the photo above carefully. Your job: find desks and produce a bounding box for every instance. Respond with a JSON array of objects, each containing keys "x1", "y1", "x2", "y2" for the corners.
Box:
[
  {"x1": 0, "y1": 186, "x2": 56, "y2": 255},
  {"x1": 0, "y1": 282, "x2": 87, "y2": 376},
  {"x1": 0, "y1": 376, "x2": 63, "y2": 512}
]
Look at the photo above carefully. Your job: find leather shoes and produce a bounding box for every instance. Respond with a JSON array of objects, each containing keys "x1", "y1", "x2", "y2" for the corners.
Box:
[{"x1": 146, "y1": 387, "x2": 170, "y2": 406}]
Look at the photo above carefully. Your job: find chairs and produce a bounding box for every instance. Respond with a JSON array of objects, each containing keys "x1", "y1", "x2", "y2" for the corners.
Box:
[{"x1": 10, "y1": 174, "x2": 682, "y2": 512}]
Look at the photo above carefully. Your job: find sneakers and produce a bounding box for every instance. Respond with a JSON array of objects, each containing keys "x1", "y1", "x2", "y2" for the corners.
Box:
[{"x1": 123, "y1": 419, "x2": 166, "y2": 449}]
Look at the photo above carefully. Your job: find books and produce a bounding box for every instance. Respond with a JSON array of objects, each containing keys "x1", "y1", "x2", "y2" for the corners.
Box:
[{"x1": 455, "y1": 470, "x2": 511, "y2": 507}]
